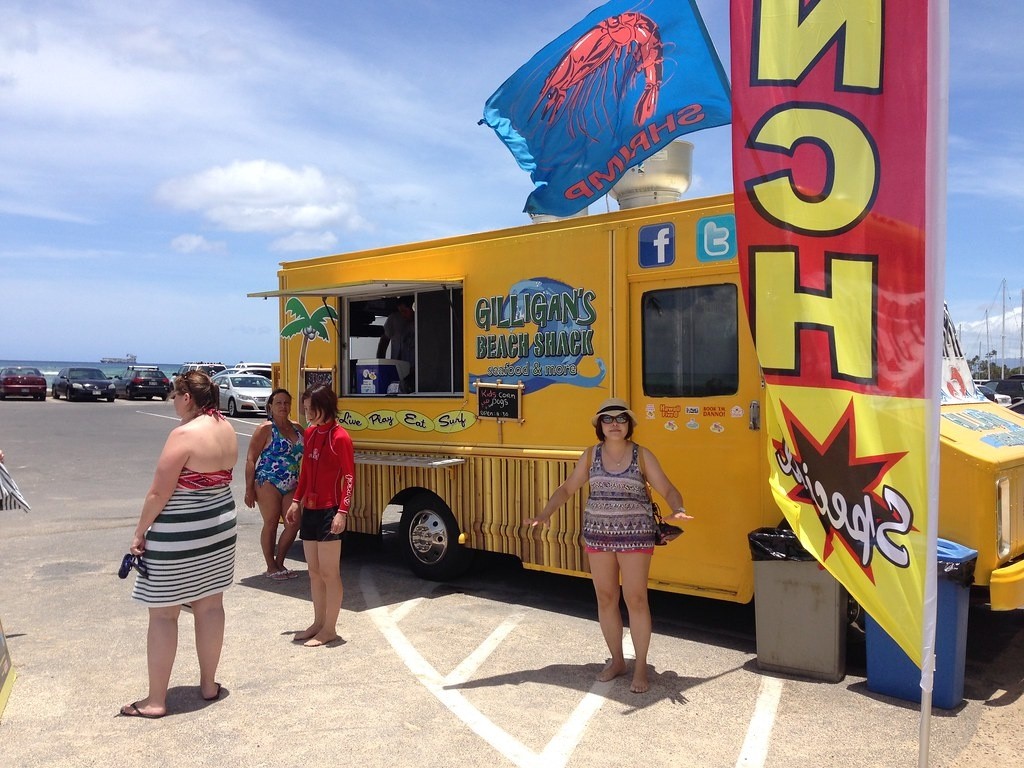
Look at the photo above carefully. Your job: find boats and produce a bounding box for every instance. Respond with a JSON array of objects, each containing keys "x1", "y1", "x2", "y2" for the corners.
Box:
[{"x1": 100, "y1": 353, "x2": 137, "y2": 363}]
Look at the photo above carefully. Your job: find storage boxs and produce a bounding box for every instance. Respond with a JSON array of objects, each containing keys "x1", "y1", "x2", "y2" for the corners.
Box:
[{"x1": 354, "y1": 359, "x2": 411, "y2": 395}]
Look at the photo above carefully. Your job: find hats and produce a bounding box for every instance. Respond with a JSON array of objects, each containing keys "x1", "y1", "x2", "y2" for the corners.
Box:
[{"x1": 591, "y1": 398, "x2": 637, "y2": 427}]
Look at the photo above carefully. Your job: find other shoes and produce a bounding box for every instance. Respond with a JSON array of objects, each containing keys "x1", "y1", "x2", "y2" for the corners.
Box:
[{"x1": 654, "y1": 522, "x2": 684, "y2": 546}]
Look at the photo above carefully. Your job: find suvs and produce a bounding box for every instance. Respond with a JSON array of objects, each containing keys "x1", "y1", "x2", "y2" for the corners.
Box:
[
  {"x1": 168, "y1": 361, "x2": 227, "y2": 391},
  {"x1": 209, "y1": 362, "x2": 272, "y2": 379}
]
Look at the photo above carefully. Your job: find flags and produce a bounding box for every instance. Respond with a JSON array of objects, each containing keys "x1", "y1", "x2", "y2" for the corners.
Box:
[{"x1": 476, "y1": 0, "x2": 733, "y2": 218}]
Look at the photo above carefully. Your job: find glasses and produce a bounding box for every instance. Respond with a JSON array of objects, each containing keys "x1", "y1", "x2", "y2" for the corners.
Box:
[
  {"x1": 181, "y1": 371, "x2": 193, "y2": 394},
  {"x1": 600, "y1": 415, "x2": 627, "y2": 424}
]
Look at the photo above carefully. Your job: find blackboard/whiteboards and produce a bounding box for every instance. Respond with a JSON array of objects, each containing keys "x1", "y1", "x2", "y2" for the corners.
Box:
[
  {"x1": 479, "y1": 386, "x2": 518, "y2": 418},
  {"x1": 305, "y1": 371, "x2": 332, "y2": 393}
]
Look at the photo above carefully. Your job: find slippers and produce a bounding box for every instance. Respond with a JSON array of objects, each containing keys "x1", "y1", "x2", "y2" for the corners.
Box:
[
  {"x1": 118, "y1": 554, "x2": 149, "y2": 579},
  {"x1": 202, "y1": 682, "x2": 221, "y2": 701},
  {"x1": 266, "y1": 569, "x2": 298, "y2": 581},
  {"x1": 120, "y1": 701, "x2": 166, "y2": 718}
]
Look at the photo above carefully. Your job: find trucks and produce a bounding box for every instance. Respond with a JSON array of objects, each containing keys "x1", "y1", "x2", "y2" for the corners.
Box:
[{"x1": 248, "y1": 120, "x2": 1024, "y2": 662}]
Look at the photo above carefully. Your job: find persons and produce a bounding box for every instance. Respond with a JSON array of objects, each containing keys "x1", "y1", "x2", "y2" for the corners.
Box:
[
  {"x1": 377, "y1": 293, "x2": 462, "y2": 393},
  {"x1": 285, "y1": 384, "x2": 354, "y2": 646},
  {"x1": 244, "y1": 388, "x2": 307, "y2": 580},
  {"x1": 120, "y1": 370, "x2": 238, "y2": 716},
  {"x1": 522, "y1": 398, "x2": 694, "y2": 693}
]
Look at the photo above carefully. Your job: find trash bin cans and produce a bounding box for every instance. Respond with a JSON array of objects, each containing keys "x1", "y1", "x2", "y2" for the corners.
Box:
[
  {"x1": 748, "y1": 527, "x2": 846, "y2": 684},
  {"x1": 865, "y1": 535, "x2": 978, "y2": 709}
]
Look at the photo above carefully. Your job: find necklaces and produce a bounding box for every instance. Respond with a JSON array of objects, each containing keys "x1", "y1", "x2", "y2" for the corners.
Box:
[
  {"x1": 604, "y1": 445, "x2": 627, "y2": 466},
  {"x1": 285, "y1": 425, "x2": 289, "y2": 430}
]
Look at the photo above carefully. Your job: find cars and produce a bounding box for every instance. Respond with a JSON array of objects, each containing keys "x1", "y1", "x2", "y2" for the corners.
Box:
[
  {"x1": 210, "y1": 374, "x2": 273, "y2": 417},
  {"x1": 974, "y1": 375, "x2": 1024, "y2": 414},
  {"x1": 113, "y1": 369, "x2": 170, "y2": 401},
  {"x1": 52, "y1": 368, "x2": 116, "y2": 402},
  {"x1": 0, "y1": 367, "x2": 47, "y2": 401}
]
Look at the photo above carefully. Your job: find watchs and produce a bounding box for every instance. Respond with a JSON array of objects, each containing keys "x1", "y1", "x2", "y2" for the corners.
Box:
[{"x1": 675, "y1": 507, "x2": 686, "y2": 513}]
